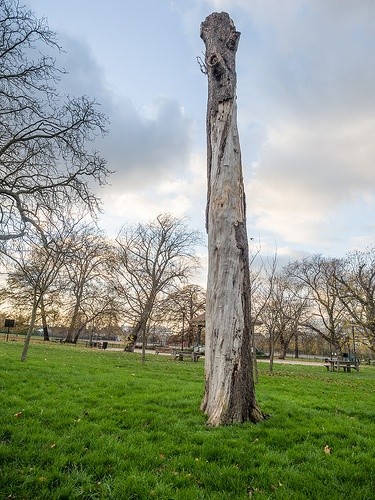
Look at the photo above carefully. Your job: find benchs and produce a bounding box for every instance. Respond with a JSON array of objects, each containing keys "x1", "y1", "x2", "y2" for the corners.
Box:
[
  {"x1": 173, "y1": 349, "x2": 202, "y2": 362},
  {"x1": 326, "y1": 359, "x2": 362, "y2": 374}
]
